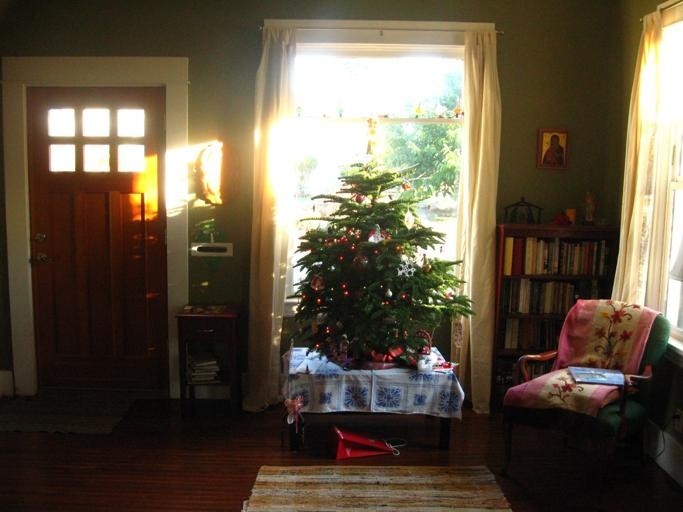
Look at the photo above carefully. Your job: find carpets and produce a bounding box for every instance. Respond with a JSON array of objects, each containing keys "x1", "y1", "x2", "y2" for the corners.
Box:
[{"x1": 242, "y1": 460, "x2": 515, "y2": 512}]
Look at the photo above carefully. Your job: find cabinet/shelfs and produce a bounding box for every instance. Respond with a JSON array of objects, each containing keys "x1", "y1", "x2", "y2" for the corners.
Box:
[{"x1": 496, "y1": 221, "x2": 620, "y2": 404}]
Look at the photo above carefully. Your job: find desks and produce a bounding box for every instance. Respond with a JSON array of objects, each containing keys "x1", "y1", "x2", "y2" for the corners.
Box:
[
  {"x1": 175, "y1": 301, "x2": 245, "y2": 420},
  {"x1": 282, "y1": 344, "x2": 460, "y2": 452}
]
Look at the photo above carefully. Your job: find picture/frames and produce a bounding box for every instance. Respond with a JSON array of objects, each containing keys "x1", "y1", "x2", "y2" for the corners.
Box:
[{"x1": 536, "y1": 127, "x2": 571, "y2": 172}]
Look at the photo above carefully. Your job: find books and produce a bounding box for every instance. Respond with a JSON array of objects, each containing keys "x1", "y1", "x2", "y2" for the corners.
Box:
[
  {"x1": 498, "y1": 361, "x2": 550, "y2": 391},
  {"x1": 184, "y1": 305, "x2": 223, "y2": 314},
  {"x1": 192, "y1": 359, "x2": 220, "y2": 380},
  {"x1": 567, "y1": 365, "x2": 625, "y2": 387},
  {"x1": 497, "y1": 236, "x2": 613, "y2": 357}
]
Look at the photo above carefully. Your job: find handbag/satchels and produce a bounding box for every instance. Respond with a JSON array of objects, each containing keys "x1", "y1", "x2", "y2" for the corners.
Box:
[{"x1": 329, "y1": 424, "x2": 392, "y2": 460}]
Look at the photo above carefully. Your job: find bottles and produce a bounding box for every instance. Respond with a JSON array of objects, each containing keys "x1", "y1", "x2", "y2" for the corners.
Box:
[{"x1": 583, "y1": 191, "x2": 596, "y2": 224}]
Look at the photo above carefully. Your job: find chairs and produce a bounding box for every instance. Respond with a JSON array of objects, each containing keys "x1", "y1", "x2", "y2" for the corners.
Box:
[{"x1": 500, "y1": 297, "x2": 671, "y2": 478}]
